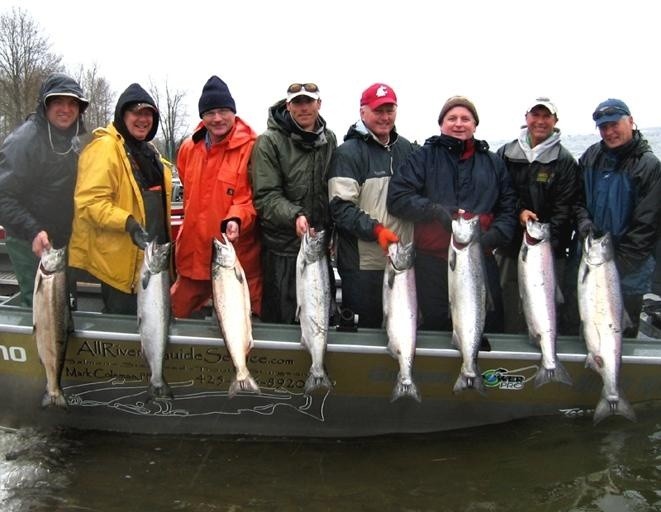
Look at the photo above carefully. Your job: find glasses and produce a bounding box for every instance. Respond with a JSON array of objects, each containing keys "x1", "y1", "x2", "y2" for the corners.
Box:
[
  {"x1": 593, "y1": 107, "x2": 628, "y2": 121},
  {"x1": 287, "y1": 82, "x2": 318, "y2": 92}
]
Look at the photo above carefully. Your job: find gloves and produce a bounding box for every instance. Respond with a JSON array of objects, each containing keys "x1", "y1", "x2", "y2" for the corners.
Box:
[
  {"x1": 431, "y1": 204, "x2": 461, "y2": 233},
  {"x1": 580, "y1": 222, "x2": 606, "y2": 240},
  {"x1": 127, "y1": 217, "x2": 150, "y2": 250},
  {"x1": 375, "y1": 225, "x2": 400, "y2": 251}
]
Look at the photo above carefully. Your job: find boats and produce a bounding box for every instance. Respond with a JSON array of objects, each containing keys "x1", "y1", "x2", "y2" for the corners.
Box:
[{"x1": 0, "y1": 236, "x2": 656, "y2": 442}]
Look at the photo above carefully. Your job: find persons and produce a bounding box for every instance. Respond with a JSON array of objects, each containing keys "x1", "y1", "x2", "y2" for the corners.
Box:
[
  {"x1": 166, "y1": 76, "x2": 263, "y2": 319},
  {"x1": 495, "y1": 97, "x2": 578, "y2": 336},
  {"x1": 571, "y1": 98, "x2": 661, "y2": 338},
  {"x1": 387, "y1": 95, "x2": 518, "y2": 331},
  {"x1": 247, "y1": 82, "x2": 338, "y2": 326},
  {"x1": 326, "y1": 84, "x2": 420, "y2": 329},
  {"x1": 67, "y1": 84, "x2": 173, "y2": 314},
  {"x1": 0, "y1": 74, "x2": 90, "y2": 306}
]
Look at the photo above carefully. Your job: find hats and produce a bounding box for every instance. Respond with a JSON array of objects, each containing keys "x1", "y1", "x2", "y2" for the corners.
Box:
[
  {"x1": 286, "y1": 81, "x2": 320, "y2": 103},
  {"x1": 525, "y1": 97, "x2": 557, "y2": 117},
  {"x1": 126, "y1": 102, "x2": 157, "y2": 113},
  {"x1": 438, "y1": 96, "x2": 479, "y2": 125},
  {"x1": 360, "y1": 84, "x2": 397, "y2": 110},
  {"x1": 199, "y1": 75, "x2": 236, "y2": 118},
  {"x1": 592, "y1": 99, "x2": 629, "y2": 126}
]
